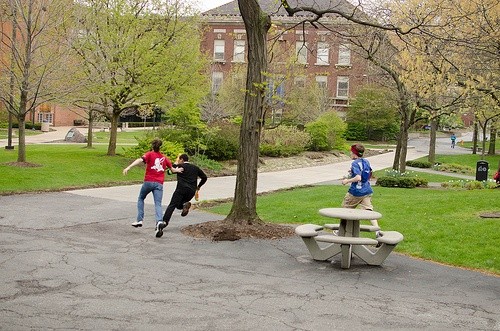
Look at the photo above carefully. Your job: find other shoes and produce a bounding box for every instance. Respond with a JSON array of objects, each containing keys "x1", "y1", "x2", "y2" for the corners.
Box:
[
  {"x1": 333, "y1": 230, "x2": 339, "y2": 236},
  {"x1": 181, "y1": 202, "x2": 191, "y2": 216},
  {"x1": 131, "y1": 221, "x2": 143, "y2": 227},
  {"x1": 155, "y1": 221, "x2": 166, "y2": 237}
]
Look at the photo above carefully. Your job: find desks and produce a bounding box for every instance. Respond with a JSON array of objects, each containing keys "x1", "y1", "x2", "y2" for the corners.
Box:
[{"x1": 317, "y1": 207, "x2": 382, "y2": 268}]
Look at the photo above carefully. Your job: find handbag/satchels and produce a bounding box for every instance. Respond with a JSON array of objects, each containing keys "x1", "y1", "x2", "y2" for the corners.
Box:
[{"x1": 360, "y1": 159, "x2": 370, "y2": 183}]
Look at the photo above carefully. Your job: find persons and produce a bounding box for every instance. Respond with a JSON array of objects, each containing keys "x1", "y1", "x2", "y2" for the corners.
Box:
[
  {"x1": 154, "y1": 153, "x2": 207, "y2": 231},
  {"x1": 123, "y1": 138, "x2": 184, "y2": 237},
  {"x1": 451, "y1": 133, "x2": 455, "y2": 148},
  {"x1": 333, "y1": 143, "x2": 381, "y2": 236}
]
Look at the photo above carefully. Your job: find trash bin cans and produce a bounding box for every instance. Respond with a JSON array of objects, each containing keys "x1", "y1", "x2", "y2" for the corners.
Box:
[
  {"x1": 475, "y1": 161, "x2": 489, "y2": 182},
  {"x1": 41, "y1": 122, "x2": 49, "y2": 132}
]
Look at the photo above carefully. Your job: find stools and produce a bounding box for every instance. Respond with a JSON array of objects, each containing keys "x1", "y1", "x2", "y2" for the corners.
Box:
[
  {"x1": 352, "y1": 230, "x2": 403, "y2": 267},
  {"x1": 295, "y1": 223, "x2": 342, "y2": 262},
  {"x1": 324, "y1": 221, "x2": 381, "y2": 233},
  {"x1": 314, "y1": 234, "x2": 379, "y2": 271}
]
